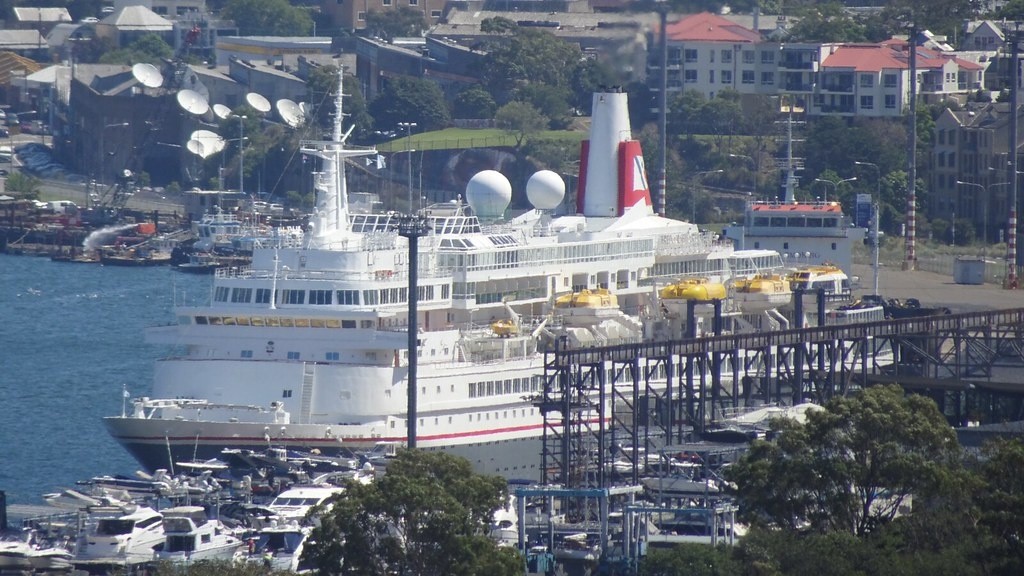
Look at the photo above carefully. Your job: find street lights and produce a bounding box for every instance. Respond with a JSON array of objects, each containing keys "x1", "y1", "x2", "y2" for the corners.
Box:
[
  {"x1": 692, "y1": 169, "x2": 724, "y2": 224},
  {"x1": 220, "y1": 137, "x2": 248, "y2": 192},
  {"x1": 389, "y1": 149, "x2": 416, "y2": 211},
  {"x1": 375, "y1": 130, "x2": 396, "y2": 210},
  {"x1": 814, "y1": 177, "x2": 857, "y2": 202},
  {"x1": 396, "y1": 122, "x2": 419, "y2": 214},
  {"x1": 729, "y1": 153, "x2": 757, "y2": 201},
  {"x1": 956, "y1": 180, "x2": 1012, "y2": 260},
  {"x1": 232, "y1": 114, "x2": 247, "y2": 193},
  {"x1": 392, "y1": 210, "x2": 437, "y2": 451},
  {"x1": 854, "y1": 162, "x2": 881, "y2": 296},
  {"x1": 25, "y1": 92, "x2": 44, "y2": 145},
  {"x1": 8, "y1": 109, "x2": 38, "y2": 175},
  {"x1": 901, "y1": 19, "x2": 933, "y2": 270},
  {"x1": 99, "y1": 122, "x2": 132, "y2": 202}
]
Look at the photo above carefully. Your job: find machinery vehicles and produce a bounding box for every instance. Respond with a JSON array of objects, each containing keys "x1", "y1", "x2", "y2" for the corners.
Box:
[{"x1": 86, "y1": 25, "x2": 203, "y2": 228}]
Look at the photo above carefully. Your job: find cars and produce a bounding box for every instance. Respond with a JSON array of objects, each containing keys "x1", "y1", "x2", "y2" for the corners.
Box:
[{"x1": 0, "y1": 105, "x2": 107, "y2": 217}]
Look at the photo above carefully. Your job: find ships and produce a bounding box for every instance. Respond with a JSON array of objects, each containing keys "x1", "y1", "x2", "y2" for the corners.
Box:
[{"x1": 101, "y1": 46, "x2": 905, "y2": 485}]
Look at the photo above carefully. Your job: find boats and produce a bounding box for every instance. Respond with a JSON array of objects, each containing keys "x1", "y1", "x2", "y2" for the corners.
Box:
[
  {"x1": 90, "y1": 191, "x2": 475, "y2": 275},
  {"x1": 490, "y1": 319, "x2": 519, "y2": 335},
  {"x1": 0, "y1": 421, "x2": 912, "y2": 576},
  {"x1": 553, "y1": 283, "x2": 624, "y2": 326},
  {"x1": 716, "y1": 94, "x2": 870, "y2": 284},
  {"x1": 785, "y1": 263, "x2": 853, "y2": 312},
  {"x1": 730, "y1": 269, "x2": 793, "y2": 313},
  {"x1": 659, "y1": 276, "x2": 728, "y2": 315}
]
